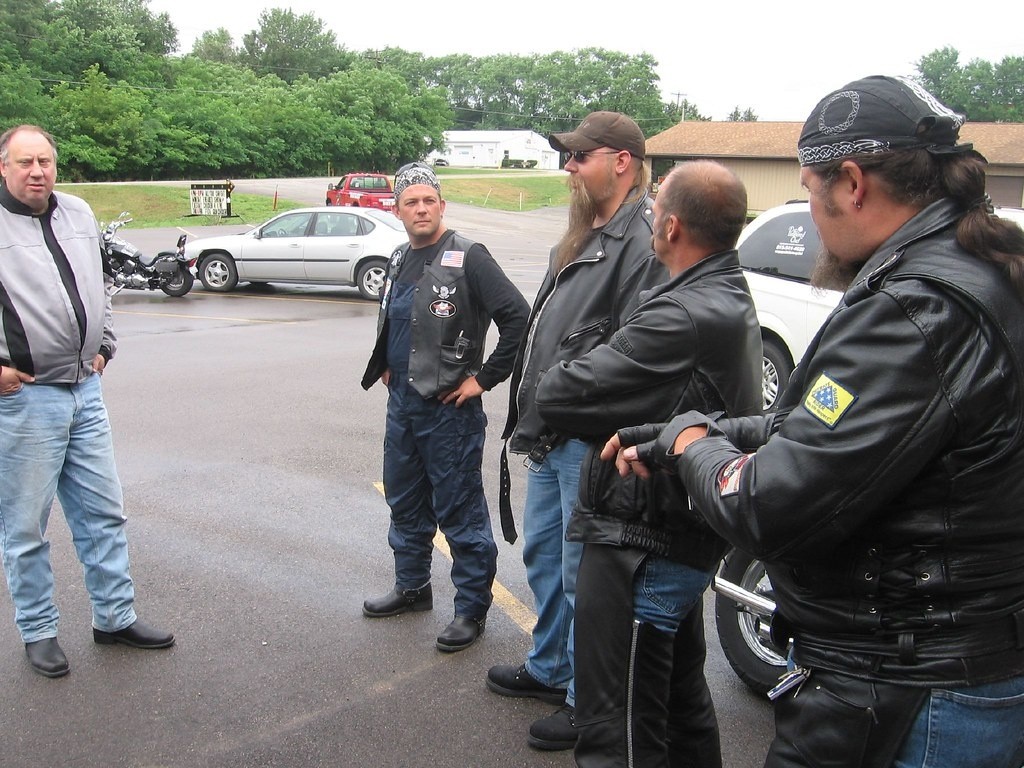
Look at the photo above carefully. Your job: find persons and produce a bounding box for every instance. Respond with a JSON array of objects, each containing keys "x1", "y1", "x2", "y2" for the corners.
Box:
[
  {"x1": 485, "y1": 110, "x2": 672, "y2": 750},
  {"x1": 535, "y1": 159, "x2": 764, "y2": 768},
  {"x1": 600, "y1": 74, "x2": 1024, "y2": 768},
  {"x1": 359, "y1": 163, "x2": 531, "y2": 653},
  {"x1": 0, "y1": 123, "x2": 175, "y2": 678}
]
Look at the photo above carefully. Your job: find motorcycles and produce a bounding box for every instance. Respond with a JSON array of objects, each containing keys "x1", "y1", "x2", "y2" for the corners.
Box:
[{"x1": 100, "y1": 210, "x2": 199, "y2": 298}]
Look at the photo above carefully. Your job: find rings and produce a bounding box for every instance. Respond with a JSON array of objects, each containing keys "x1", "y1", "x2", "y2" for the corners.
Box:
[{"x1": 453, "y1": 392, "x2": 456, "y2": 396}]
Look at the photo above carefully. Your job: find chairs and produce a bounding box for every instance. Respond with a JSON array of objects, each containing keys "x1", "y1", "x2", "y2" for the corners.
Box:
[
  {"x1": 316, "y1": 221, "x2": 327, "y2": 233},
  {"x1": 352, "y1": 181, "x2": 360, "y2": 187},
  {"x1": 374, "y1": 181, "x2": 383, "y2": 188},
  {"x1": 330, "y1": 216, "x2": 352, "y2": 234}
]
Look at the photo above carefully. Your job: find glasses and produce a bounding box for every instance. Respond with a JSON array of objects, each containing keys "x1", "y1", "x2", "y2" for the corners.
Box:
[{"x1": 565, "y1": 150, "x2": 631, "y2": 164}]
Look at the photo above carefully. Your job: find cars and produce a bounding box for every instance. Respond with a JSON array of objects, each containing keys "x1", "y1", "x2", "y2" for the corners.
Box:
[
  {"x1": 435, "y1": 157, "x2": 450, "y2": 167},
  {"x1": 177, "y1": 206, "x2": 410, "y2": 302},
  {"x1": 736, "y1": 199, "x2": 1024, "y2": 411}
]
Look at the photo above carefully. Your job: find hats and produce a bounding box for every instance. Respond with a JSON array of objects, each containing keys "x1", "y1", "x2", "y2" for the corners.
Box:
[{"x1": 548, "y1": 111, "x2": 645, "y2": 160}]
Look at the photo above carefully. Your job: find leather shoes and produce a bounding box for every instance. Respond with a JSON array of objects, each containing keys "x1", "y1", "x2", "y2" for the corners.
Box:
[
  {"x1": 486, "y1": 662, "x2": 566, "y2": 706},
  {"x1": 528, "y1": 697, "x2": 579, "y2": 749},
  {"x1": 93, "y1": 619, "x2": 175, "y2": 649},
  {"x1": 436, "y1": 615, "x2": 486, "y2": 651},
  {"x1": 362, "y1": 584, "x2": 432, "y2": 617},
  {"x1": 26, "y1": 636, "x2": 70, "y2": 678}
]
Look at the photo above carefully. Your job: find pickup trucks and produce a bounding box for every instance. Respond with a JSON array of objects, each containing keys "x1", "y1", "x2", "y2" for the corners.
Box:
[{"x1": 326, "y1": 172, "x2": 397, "y2": 212}]
